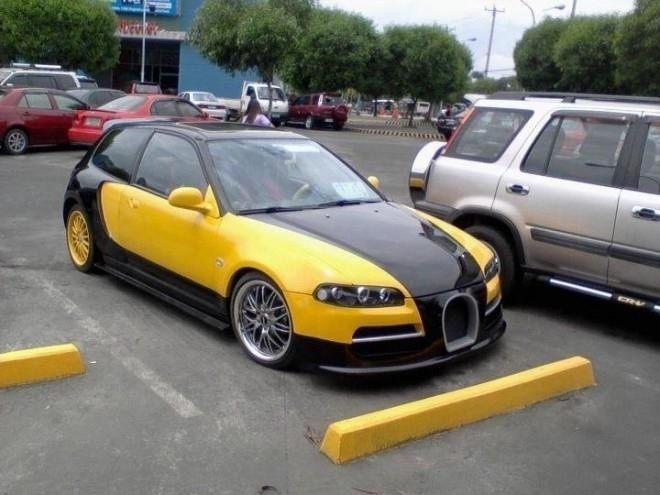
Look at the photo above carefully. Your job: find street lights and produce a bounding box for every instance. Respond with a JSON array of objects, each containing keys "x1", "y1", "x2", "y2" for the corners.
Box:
[{"x1": 520, "y1": 0, "x2": 565, "y2": 27}]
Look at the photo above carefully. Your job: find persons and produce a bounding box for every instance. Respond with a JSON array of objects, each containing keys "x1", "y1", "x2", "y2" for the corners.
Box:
[
  {"x1": 445, "y1": 103, "x2": 451, "y2": 116},
  {"x1": 236, "y1": 99, "x2": 276, "y2": 128}
]
